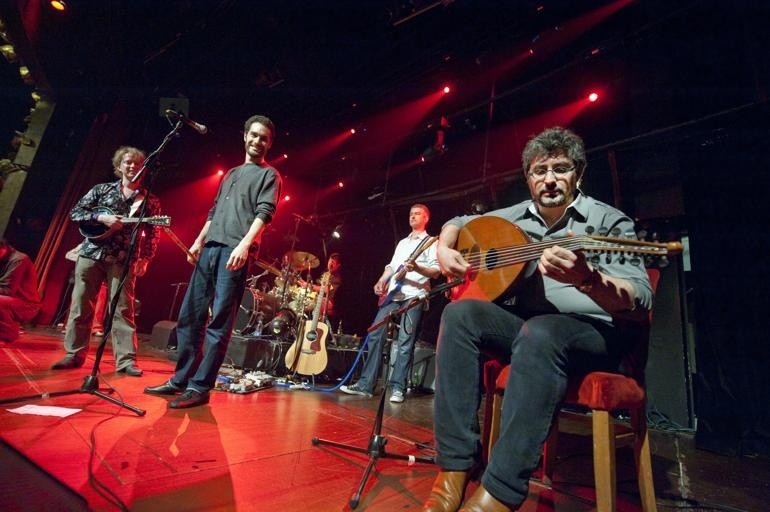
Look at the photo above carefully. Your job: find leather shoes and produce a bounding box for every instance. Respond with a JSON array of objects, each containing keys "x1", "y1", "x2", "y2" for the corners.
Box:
[
  {"x1": 144, "y1": 381, "x2": 183, "y2": 395},
  {"x1": 422, "y1": 470, "x2": 467, "y2": 512},
  {"x1": 52, "y1": 357, "x2": 78, "y2": 368},
  {"x1": 123, "y1": 366, "x2": 142, "y2": 376},
  {"x1": 169, "y1": 390, "x2": 209, "y2": 407},
  {"x1": 458, "y1": 485, "x2": 511, "y2": 512}
]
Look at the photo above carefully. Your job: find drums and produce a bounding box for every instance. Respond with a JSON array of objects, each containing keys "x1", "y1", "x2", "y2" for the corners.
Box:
[
  {"x1": 232, "y1": 287, "x2": 281, "y2": 336},
  {"x1": 296, "y1": 286, "x2": 318, "y2": 311},
  {"x1": 275, "y1": 278, "x2": 297, "y2": 301}
]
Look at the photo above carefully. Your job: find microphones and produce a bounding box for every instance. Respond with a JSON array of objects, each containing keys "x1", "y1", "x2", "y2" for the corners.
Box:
[
  {"x1": 160, "y1": 107, "x2": 207, "y2": 136},
  {"x1": 12, "y1": 128, "x2": 36, "y2": 149},
  {"x1": 418, "y1": 276, "x2": 466, "y2": 304}
]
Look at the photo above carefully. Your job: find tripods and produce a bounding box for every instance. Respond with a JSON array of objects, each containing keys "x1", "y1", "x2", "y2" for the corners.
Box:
[
  {"x1": 252, "y1": 238, "x2": 297, "y2": 342},
  {"x1": 0, "y1": 129, "x2": 183, "y2": 419},
  {"x1": 315, "y1": 243, "x2": 336, "y2": 344},
  {"x1": 312, "y1": 295, "x2": 437, "y2": 510}
]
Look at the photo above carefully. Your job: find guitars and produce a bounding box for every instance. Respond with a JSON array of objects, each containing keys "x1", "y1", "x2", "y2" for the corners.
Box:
[
  {"x1": 285, "y1": 271, "x2": 330, "y2": 376},
  {"x1": 378, "y1": 235, "x2": 441, "y2": 308}
]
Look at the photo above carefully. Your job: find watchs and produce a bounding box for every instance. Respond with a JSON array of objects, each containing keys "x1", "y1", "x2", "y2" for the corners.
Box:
[{"x1": 576, "y1": 265, "x2": 598, "y2": 294}]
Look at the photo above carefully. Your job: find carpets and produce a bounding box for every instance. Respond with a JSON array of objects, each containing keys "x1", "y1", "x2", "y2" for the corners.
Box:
[{"x1": 0, "y1": 330, "x2": 646, "y2": 512}]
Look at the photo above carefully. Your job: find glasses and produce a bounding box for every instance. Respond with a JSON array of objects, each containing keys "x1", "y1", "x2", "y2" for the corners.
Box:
[{"x1": 528, "y1": 165, "x2": 577, "y2": 179}]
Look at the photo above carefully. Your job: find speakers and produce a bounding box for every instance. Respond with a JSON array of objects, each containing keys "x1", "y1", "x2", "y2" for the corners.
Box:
[
  {"x1": 151, "y1": 319, "x2": 177, "y2": 350},
  {"x1": 644, "y1": 241, "x2": 696, "y2": 434}
]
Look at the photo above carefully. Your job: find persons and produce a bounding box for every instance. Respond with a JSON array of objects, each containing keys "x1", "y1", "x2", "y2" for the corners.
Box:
[
  {"x1": 145, "y1": 114, "x2": 282, "y2": 408},
  {"x1": 422, "y1": 126, "x2": 656, "y2": 511},
  {"x1": 301, "y1": 251, "x2": 344, "y2": 319},
  {"x1": 50, "y1": 144, "x2": 162, "y2": 378},
  {"x1": 338, "y1": 203, "x2": 442, "y2": 405},
  {"x1": 0, "y1": 239, "x2": 42, "y2": 345}
]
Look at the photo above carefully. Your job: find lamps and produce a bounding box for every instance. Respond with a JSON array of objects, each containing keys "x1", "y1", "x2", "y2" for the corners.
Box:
[{"x1": 0, "y1": 43, "x2": 48, "y2": 102}]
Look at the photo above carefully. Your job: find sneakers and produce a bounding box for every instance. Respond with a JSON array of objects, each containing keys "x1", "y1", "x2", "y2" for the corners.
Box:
[
  {"x1": 340, "y1": 383, "x2": 373, "y2": 397},
  {"x1": 390, "y1": 391, "x2": 404, "y2": 402}
]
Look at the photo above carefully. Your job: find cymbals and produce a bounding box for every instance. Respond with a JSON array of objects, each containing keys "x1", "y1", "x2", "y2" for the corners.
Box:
[
  {"x1": 254, "y1": 261, "x2": 281, "y2": 276},
  {"x1": 284, "y1": 251, "x2": 320, "y2": 271}
]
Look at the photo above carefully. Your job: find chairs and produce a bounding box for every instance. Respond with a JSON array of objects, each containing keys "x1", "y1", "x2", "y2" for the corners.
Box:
[{"x1": 481, "y1": 265, "x2": 660, "y2": 511}]
